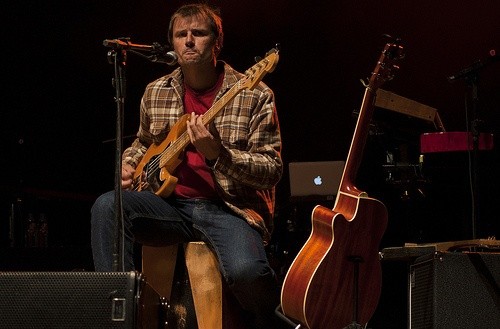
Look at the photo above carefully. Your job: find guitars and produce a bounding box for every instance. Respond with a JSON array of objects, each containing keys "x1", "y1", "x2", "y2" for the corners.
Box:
[
  {"x1": 131, "y1": 44, "x2": 279, "y2": 196},
  {"x1": 281, "y1": 43, "x2": 406, "y2": 329}
]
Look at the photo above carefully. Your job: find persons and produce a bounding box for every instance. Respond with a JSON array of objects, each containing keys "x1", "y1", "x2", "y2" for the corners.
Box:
[{"x1": 86, "y1": 2, "x2": 289, "y2": 329}]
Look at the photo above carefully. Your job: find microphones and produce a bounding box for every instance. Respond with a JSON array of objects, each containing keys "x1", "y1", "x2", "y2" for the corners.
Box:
[
  {"x1": 447, "y1": 58, "x2": 484, "y2": 80},
  {"x1": 150, "y1": 51, "x2": 178, "y2": 66}
]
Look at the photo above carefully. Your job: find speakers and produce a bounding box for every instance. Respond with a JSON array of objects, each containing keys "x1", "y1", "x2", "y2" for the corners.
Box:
[
  {"x1": 409, "y1": 252, "x2": 500, "y2": 329},
  {"x1": 0, "y1": 270, "x2": 163, "y2": 328}
]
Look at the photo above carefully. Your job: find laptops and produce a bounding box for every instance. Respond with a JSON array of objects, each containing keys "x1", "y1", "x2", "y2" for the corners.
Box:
[{"x1": 289, "y1": 162, "x2": 346, "y2": 198}]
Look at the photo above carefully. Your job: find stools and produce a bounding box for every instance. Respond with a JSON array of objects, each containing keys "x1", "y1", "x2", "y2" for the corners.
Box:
[{"x1": 137, "y1": 239, "x2": 227, "y2": 329}]
